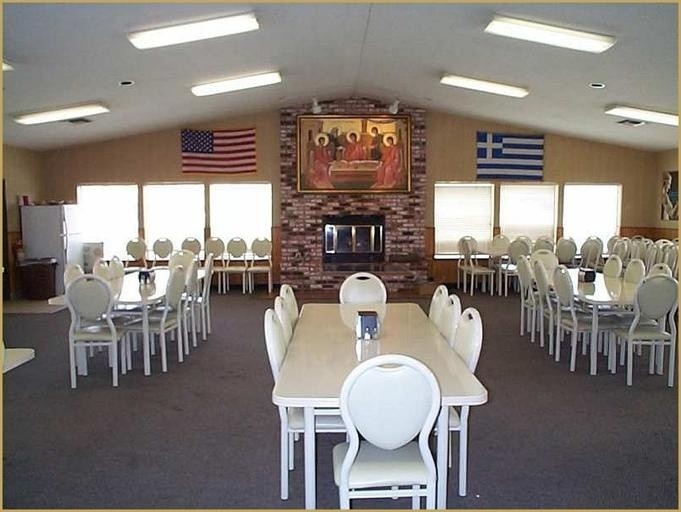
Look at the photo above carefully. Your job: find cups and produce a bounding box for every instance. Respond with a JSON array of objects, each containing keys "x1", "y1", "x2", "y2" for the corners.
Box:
[{"x1": 23, "y1": 195, "x2": 29, "y2": 206}]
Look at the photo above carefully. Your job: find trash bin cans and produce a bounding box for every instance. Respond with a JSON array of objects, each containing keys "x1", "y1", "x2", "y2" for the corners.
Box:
[{"x1": 16, "y1": 257, "x2": 58, "y2": 302}]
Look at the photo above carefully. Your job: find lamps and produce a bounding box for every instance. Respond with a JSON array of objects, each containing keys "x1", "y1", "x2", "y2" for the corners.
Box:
[
  {"x1": 190, "y1": 70, "x2": 282, "y2": 98},
  {"x1": 604, "y1": 104, "x2": 679, "y2": 127},
  {"x1": 440, "y1": 74, "x2": 529, "y2": 99},
  {"x1": 13, "y1": 102, "x2": 112, "y2": 126},
  {"x1": 483, "y1": 14, "x2": 617, "y2": 55},
  {"x1": 126, "y1": 12, "x2": 260, "y2": 50}
]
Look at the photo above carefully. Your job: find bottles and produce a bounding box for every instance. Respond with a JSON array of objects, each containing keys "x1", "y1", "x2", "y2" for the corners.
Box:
[
  {"x1": 372, "y1": 328, "x2": 379, "y2": 339},
  {"x1": 18, "y1": 194, "x2": 24, "y2": 206},
  {"x1": 364, "y1": 327, "x2": 371, "y2": 341}
]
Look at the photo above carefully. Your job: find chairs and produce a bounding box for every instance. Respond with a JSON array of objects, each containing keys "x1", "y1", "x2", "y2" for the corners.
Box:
[
  {"x1": 428, "y1": 285, "x2": 462, "y2": 346},
  {"x1": 340, "y1": 272, "x2": 388, "y2": 303},
  {"x1": 332, "y1": 351, "x2": 441, "y2": 511},
  {"x1": 63, "y1": 237, "x2": 274, "y2": 391},
  {"x1": 264, "y1": 307, "x2": 349, "y2": 500},
  {"x1": 457, "y1": 234, "x2": 678, "y2": 388},
  {"x1": 275, "y1": 283, "x2": 299, "y2": 348},
  {"x1": 433, "y1": 307, "x2": 483, "y2": 497}
]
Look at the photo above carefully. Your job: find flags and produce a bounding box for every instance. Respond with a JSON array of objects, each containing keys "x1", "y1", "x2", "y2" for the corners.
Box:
[
  {"x1": 476, "y1": 131, "x2": 544, "y2": 182},
  {"x1": 180, "y1": 128, "x2": 257, "y2": 176}
]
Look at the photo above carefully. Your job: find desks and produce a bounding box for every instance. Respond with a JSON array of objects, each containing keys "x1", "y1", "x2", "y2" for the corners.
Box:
[{"x1": 271, "y1": 303, "x2": 488, "y2": 509}]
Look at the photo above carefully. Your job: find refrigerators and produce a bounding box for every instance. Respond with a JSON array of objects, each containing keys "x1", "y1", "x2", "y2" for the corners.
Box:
[{"x1": 20, "y1": 204, "x2": 85, "y2": 298}]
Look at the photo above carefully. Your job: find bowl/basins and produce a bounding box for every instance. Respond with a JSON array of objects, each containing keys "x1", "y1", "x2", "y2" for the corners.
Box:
[
  {"x1": 65, "y1": 200, "x2": 73, "y2": 204},
  {"x1": 46, "y1": 199, "x2": 65, "y2": 204}
]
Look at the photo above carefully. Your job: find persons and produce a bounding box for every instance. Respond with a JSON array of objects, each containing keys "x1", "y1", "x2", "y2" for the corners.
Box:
[
  {"x1": 307, "y1": 126, "x2": 400, "y2": 186},
  {"x1": 661, "y1": 171, "x2": 678, "y2": 221}
]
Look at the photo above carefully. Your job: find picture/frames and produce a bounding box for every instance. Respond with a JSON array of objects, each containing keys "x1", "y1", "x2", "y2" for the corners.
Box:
[{"x1": 295, "y1": 114, "x2": 413, "y2": 194}]
